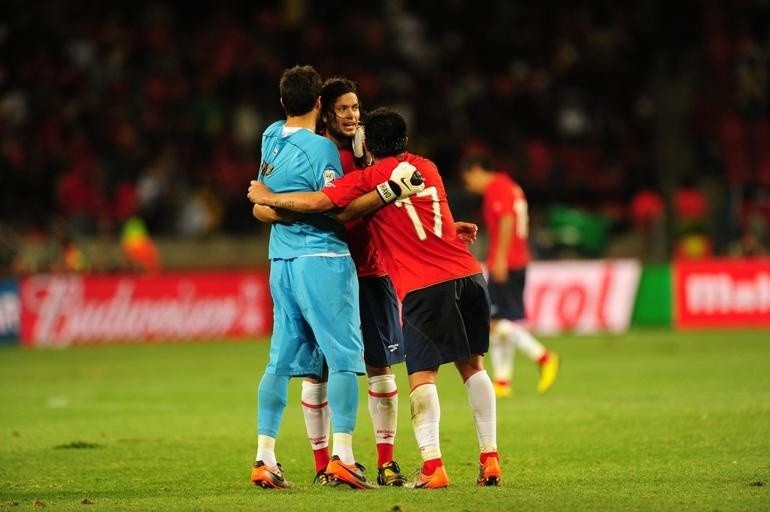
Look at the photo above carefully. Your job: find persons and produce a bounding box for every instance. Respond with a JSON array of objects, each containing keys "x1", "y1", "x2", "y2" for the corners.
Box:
[
  {"x1": 248, "y1": 106, "x2": 502, "y2": 490},
  {"x1": 253, "y1": 65, "x2": 424, "y2": 489},
  {"x1": 460, "y1": 154, "x2": 559, "y2": 397},
  {"x1": 301, "y1": 78, "x2": 478, "y2": 486}
]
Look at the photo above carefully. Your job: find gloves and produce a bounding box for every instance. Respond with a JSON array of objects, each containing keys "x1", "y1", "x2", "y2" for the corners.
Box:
[{"x1": 376, "y1": 161, "x2": 426, "y2": 205}]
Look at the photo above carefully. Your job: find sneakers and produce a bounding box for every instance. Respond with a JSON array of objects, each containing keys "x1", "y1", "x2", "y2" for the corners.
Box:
[
  {"x1": 250, "y1": 460, "x2": 295, "y2": 490},
  {"x1": 491, "y1": 381, "x2": 512, "y2": 399},
  {"x1": 412, "y1": 465, "x2": 449, "y2": 490},
  {"x1": 537, "y1": 352, "x2": 559, "y2": 394},
  {"x1": 376, "y1": 460, "x2": 408, "y2": 487},
  {"x1": 325, "y1": 454, "x2": 378, "y2": 490},
  {"x1": 476, "y1": 452, "x2": 501, "y2": 487},
  {"x1": 312, "y1": 467, "x2": 340, "y2": 487}
]
